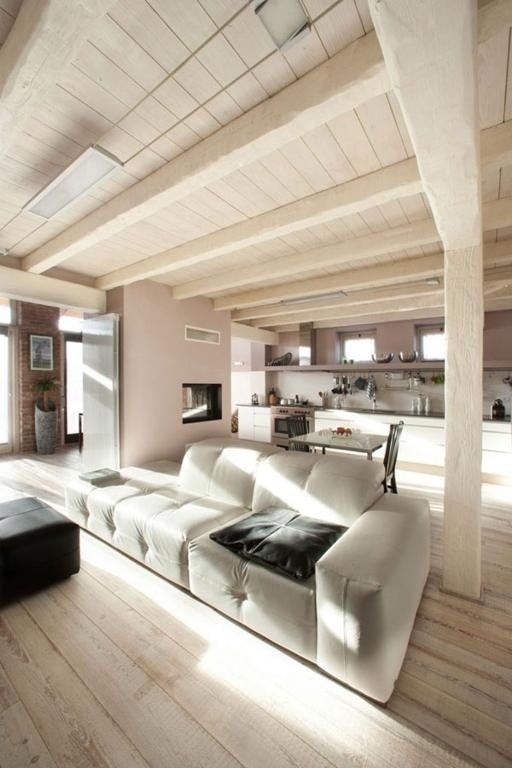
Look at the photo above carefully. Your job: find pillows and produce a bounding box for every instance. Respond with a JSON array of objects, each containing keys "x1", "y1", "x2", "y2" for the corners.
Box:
[{"x1": 209, "y1": 506, "x2": 348, "y2": 580}]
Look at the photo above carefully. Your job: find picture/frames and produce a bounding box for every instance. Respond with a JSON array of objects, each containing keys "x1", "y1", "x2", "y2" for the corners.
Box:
[{"x1": 29, "y1": 335, "x2": 54, "y2": 372}]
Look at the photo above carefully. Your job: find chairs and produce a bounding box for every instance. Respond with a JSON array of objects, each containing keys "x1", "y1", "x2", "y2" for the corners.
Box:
[
  {"x1": 380, "y1": 421, "x2": 404, "y2": 494},
  {"x1": 285, "y1": 414, "x2": 310, "y2": 453}
]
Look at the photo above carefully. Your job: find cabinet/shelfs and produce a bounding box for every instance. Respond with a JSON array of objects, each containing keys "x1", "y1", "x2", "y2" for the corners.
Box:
[
  {"x1": 313, "y1": 406, "x2": 512, "y2": 475},
  {"x1": 236, "y1": 404, "x2": 272, "y2": 443}
]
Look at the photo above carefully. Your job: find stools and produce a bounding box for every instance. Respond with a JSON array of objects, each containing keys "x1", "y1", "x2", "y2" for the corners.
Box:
[{"x1": 0, "y1": 497, "x2": 80, "y2": 611}]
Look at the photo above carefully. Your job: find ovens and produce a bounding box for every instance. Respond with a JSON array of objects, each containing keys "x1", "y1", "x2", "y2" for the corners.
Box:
[{"x1": 271, "y1": 413, "x2": 315, "y2": 446}]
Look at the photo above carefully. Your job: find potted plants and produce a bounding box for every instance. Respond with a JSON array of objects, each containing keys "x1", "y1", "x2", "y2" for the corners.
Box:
[{"x1": 31, "y1": 373, "x2": 60, "y2": 455}]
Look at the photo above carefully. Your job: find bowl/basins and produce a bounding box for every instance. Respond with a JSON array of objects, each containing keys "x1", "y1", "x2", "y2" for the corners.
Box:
[
  {"x1": 398, "y1": 350, "x2": 418, "y2": 362},
  {"x1": 371, "y1": 350, "x2": 394, "y2": 363}
]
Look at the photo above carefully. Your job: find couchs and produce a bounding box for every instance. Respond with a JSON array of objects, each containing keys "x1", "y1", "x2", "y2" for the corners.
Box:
[{"x1": 64, "y1": 437, "x2": 431, "y2": 705}]
